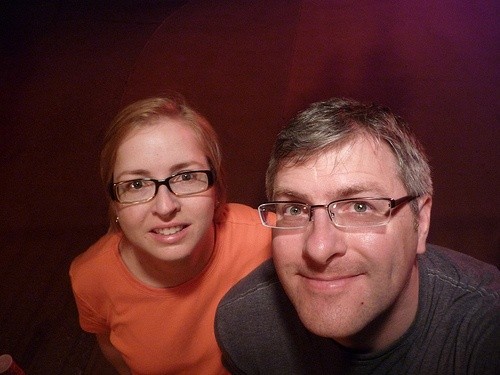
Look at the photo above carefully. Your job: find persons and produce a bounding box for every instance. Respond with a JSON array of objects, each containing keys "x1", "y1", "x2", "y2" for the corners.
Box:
[
  {"x1": 214, "y1": 98, "x2": 500, "y2": 375},
  {"x1": 69, "y1": 95, "x2": 279, "y2": 375}
]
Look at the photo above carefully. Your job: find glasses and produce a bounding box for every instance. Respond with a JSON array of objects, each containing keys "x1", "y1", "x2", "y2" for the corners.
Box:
[
  {"x1": 258, "y1": 195, "x2": 420, "y2": 229},
  {"x1": 108, "y1": 170, "x2": 214, "y2": 203}
]
